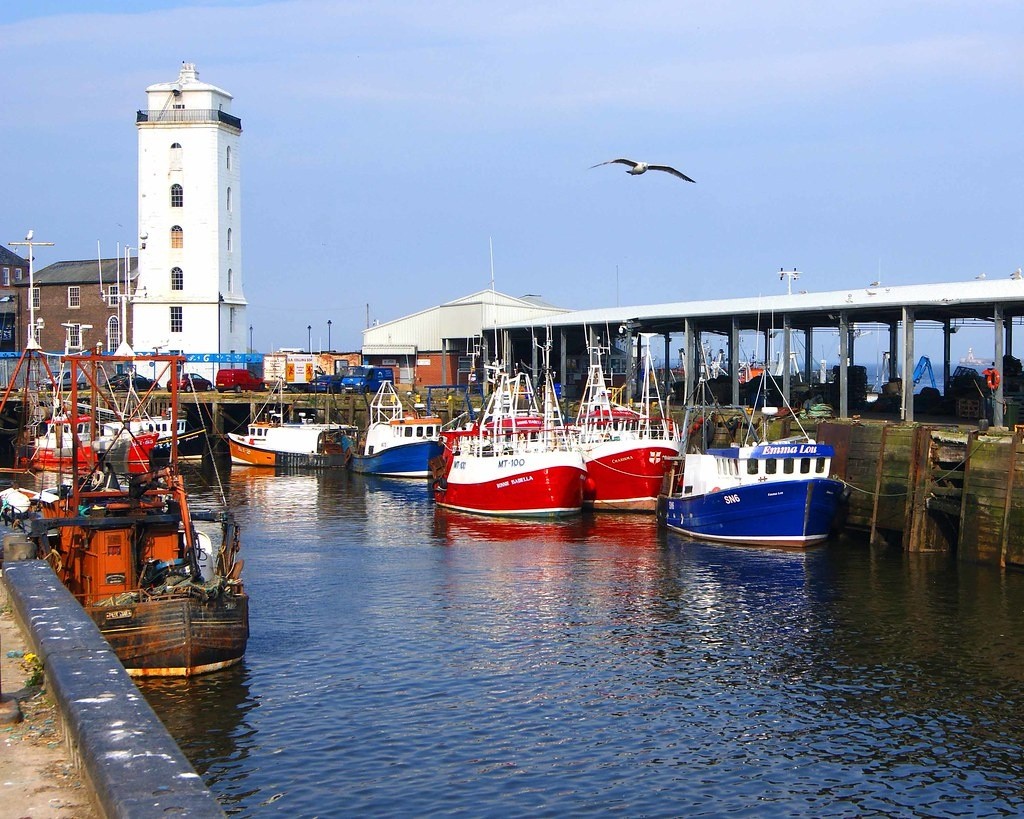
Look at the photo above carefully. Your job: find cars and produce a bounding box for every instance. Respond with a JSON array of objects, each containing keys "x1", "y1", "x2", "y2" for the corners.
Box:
[
  {"x1": 167, "y1": 373, "x2": 212, "y2": 392},
  {"x1": 106, "y1": 372, "x2": 161, "y2": 392},
  {"x1": 308, "y1": 375, "x2": 343, "y2": 393}
]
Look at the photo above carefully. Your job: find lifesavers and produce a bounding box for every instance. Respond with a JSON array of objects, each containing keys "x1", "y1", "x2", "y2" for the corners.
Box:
[
  {"x1": 987, "y1": 369, "x2": 1000, "y2": 389},
  {"x1": 430, "y1": 477, "x2": 448, "y2": 492},
  {"x1": 711, "y1": 487, "x2": 722, "y2": 493}
]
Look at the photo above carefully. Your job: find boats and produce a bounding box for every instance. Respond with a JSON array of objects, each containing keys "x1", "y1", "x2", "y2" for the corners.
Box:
[
  {"x1": 227, "y1": 361, "x2": 359, "y2": 467},
  {"x1": 339, "y1": 380, "x2": 448, "y2": 478},
  {"x1": 557, "y1": 319, "x2": 683, "y2": 512},
  {"x1": 0, "y1": 347, "x2": 250, "y2": 677},
  {"x1": 10, "y1": 243, "x2": 207, "y2": 463},
  {"x1": 656, "y1": 308, "x2": 848, "y2": 548},
  {"x1": 428, "y1": 320, "x2": 587, "y2": 518}
]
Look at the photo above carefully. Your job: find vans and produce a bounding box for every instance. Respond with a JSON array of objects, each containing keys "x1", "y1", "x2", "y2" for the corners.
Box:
[
  {"x1": 216, "y1": 368, "x2": 265, "y2": 393},
  {"x1": 36, "y1": 367, "x2": 88, "y2": 391},
  {"x1": 341, "y1": 365, "x2": 394, "y2": 394}
]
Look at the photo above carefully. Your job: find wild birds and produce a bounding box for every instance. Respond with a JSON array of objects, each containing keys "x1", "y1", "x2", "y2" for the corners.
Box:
[
  {"x1": 587, "y1": 159, "x2": 698, "y2": 184},
  {"x1": 24, "y1": 230, "x2": 34, "y2": 242}
]
